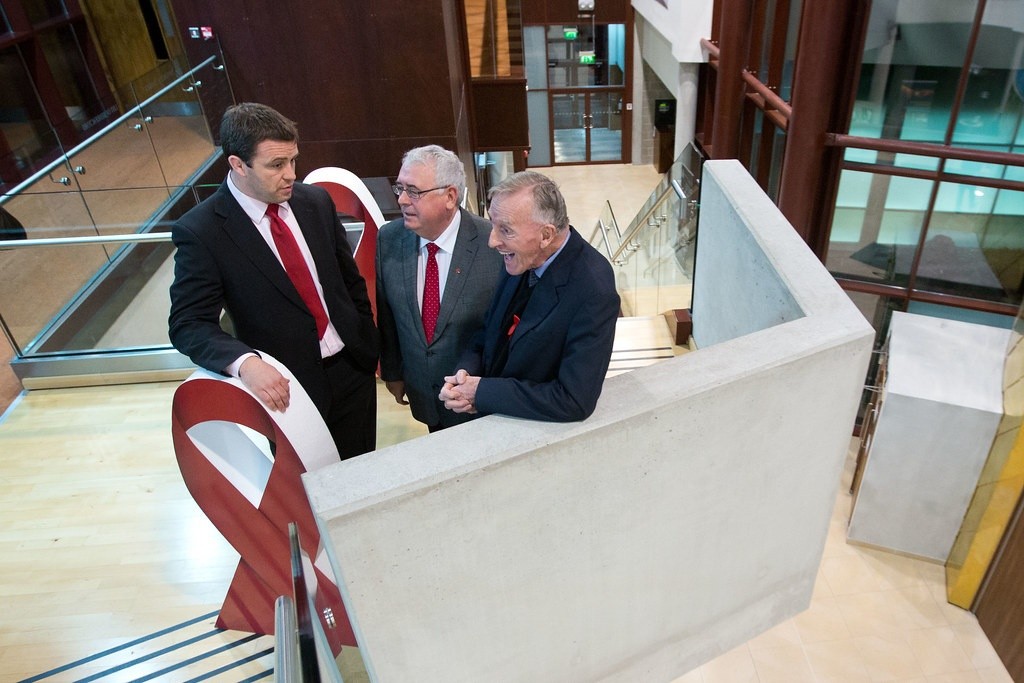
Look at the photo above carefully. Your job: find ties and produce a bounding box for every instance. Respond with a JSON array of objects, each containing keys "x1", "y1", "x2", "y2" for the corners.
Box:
[
  {"x1": 266, "y1": 203, "x2": 329, "y2": 341},
  {"x1": 528, "y1": 271, "x2": 539, "y2": 287},
  {"x1": 421, "y1": 243, "x2": 440, "y2": 344}
]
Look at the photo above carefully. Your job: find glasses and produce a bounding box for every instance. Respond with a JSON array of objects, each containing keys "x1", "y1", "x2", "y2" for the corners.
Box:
[{"x1": 391, "y1": 185, "x2": 450, "y2": 199}]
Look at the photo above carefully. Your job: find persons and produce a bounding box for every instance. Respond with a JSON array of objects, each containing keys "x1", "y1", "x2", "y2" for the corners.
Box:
[
  {"x1": 439, "y1": 171, "x2": 621, "y2": 422},
  {"x1": 169, "y1": 103, "x2": 381, "y2": 460},
  {"x1": 374, "y1": 146, "x2": 506, "y2": 434}
]
